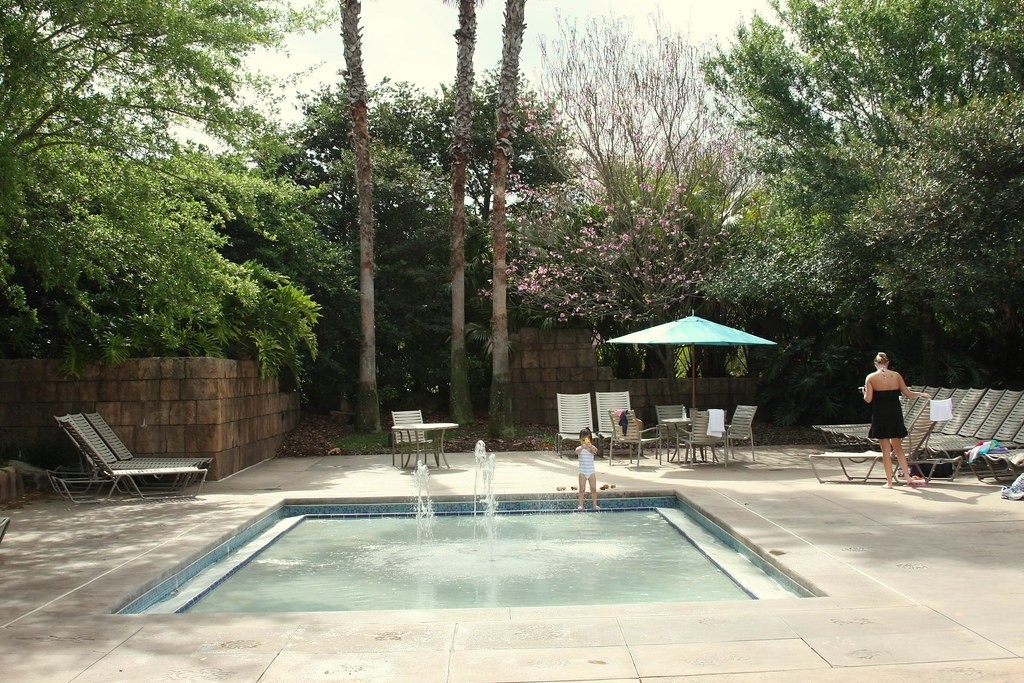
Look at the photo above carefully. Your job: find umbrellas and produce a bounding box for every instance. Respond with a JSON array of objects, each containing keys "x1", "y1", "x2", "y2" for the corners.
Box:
[{"x1": 605, "y1": 309, "x2": 778, "y2": 407}]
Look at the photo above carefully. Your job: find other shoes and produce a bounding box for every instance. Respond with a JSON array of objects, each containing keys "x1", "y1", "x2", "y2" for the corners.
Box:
[{"x1": 1001, "y1": 486, "x2": 1024, "y2": 499}]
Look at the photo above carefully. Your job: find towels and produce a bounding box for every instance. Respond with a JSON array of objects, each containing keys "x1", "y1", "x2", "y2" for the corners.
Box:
[
  {"x1": 929, "y1": 398, "x2": 954, "y2": 422},
  {"x1": 706, "y1": 408, "x2": 726, "y2": 438}
]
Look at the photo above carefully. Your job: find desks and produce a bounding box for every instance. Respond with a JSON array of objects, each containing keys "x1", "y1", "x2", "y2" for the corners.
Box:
[
  {"x1": 661, "y1": 417, "x2": 691, "y2": 463},
  {"x1": 392, "y1": 422, "x2": 459, "y2": 470}
]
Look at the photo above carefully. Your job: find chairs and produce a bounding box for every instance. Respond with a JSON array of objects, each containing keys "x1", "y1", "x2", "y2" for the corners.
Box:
[
  {"x1": 713, "y1": 404, "x2": 758, "y2": 463},
  {"x1": 45, "y1": 411, "x2": 214, "y2": 503},
  {"x1": 675, "y1": 409, "x2": 728, "y2": 468},
  {"x1": 654, "y1": 404, "x2": 692, "y2": 464},
  {"x1": 594, "y1": 390, "x2": 633, "y2": 458},
  {"x1": 392, "y1": 408, "x2": 433, "y2": 469},
  {"x1": 607, "y1": 407, "x2": 663, "y2": 469},
  {"x1": 809, "y1": 383, "x2": 1024, "y2": 488},
  {"x1": 553, "y1": 392, "x2": 603, "y2": 459}
]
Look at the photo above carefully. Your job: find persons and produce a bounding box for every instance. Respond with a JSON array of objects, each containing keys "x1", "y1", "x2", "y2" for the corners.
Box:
[
  {"x1": 862, "y1": 352, "x2": 931, "y2": 489},
  {"x1": 575, "y1": 427, "x2": 600, "y2": 510}
]
